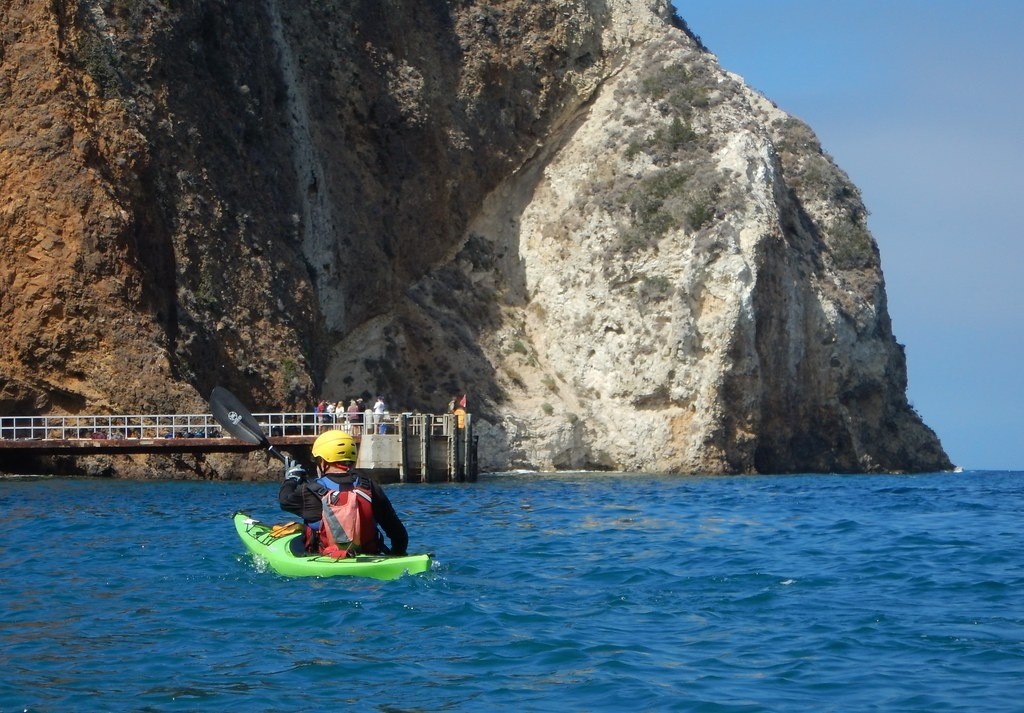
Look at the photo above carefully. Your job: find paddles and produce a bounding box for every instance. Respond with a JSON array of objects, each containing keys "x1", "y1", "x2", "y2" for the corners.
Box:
[{"x1": 208, "y1": 386, "x2": 391, "y2": 556}]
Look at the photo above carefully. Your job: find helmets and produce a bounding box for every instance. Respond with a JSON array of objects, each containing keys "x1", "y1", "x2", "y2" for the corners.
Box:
[{"x1": 312, "y1": 430, "x2": 358, "y2": 463}]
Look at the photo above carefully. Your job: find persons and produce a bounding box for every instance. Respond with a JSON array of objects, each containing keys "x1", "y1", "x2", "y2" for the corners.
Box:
[
  {"x1": 279, "y1": 430, "x2": 409, "y2": 558},
  {"x1": 311, "y1": 394, "x2": 390, "y2": 435},
  {"x1": 448, "y1": 396, "x2": 457, "y2": 414}
]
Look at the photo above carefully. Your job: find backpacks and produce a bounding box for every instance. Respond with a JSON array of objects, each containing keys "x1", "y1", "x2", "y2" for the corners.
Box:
[{"x1": 306, "y1": 477, "x2": 376, "y2": 557}]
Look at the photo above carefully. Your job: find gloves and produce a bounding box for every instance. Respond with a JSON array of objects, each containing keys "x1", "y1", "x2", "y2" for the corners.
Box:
[{"x1": 285, "y1": 459, "x2": 307, "y2": 483}]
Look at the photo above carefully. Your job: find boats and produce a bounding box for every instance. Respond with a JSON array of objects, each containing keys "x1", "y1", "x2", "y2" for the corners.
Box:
[{"x1": 233, "y1": 512, "x2": 432, "y2": 581}]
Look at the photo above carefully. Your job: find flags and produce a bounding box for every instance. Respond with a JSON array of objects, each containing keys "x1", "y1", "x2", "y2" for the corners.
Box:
[{"x1": 460, "y1": 397, "x2": 465, "y2": 407}]
